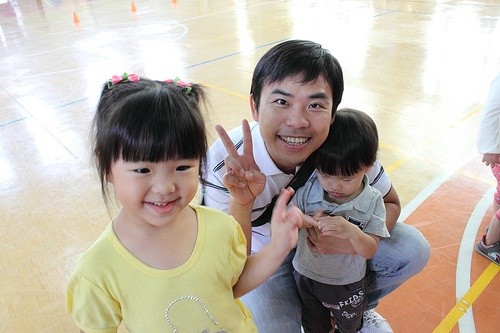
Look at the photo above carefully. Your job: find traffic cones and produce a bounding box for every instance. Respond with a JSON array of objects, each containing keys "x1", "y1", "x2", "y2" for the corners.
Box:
[
  {"x1": 125, "y1": 0, "x2": 143, "y2": 15},
  {"x1": 168, "y1": 0, "x2": 183, "y2": 6},
  {"x1": 67, "y1": 8, "x2": 83, "y2": 27}
]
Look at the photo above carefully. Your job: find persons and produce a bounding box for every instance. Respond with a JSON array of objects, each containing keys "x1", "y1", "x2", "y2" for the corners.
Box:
[
  {"x1": 477, "y1": 71, "x2": 500, "y2": 264},
  {"x1": 205, "y1": 40, "x2": 431, "y2": 333},
  {"x1": 64, "y1": 73, "x2": 304, "y2": 333},
  {"x1": 285, "y1": 109, "x2": 390, "y2": 333}
]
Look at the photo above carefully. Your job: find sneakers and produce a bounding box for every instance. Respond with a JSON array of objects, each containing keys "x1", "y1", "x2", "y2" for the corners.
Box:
[
  {"x1": 357, "y1": 308, "x2": 394, "y2": 333},
  {"x1": 475, "y1": 226, "x2": 500, "y2": 267}
]
